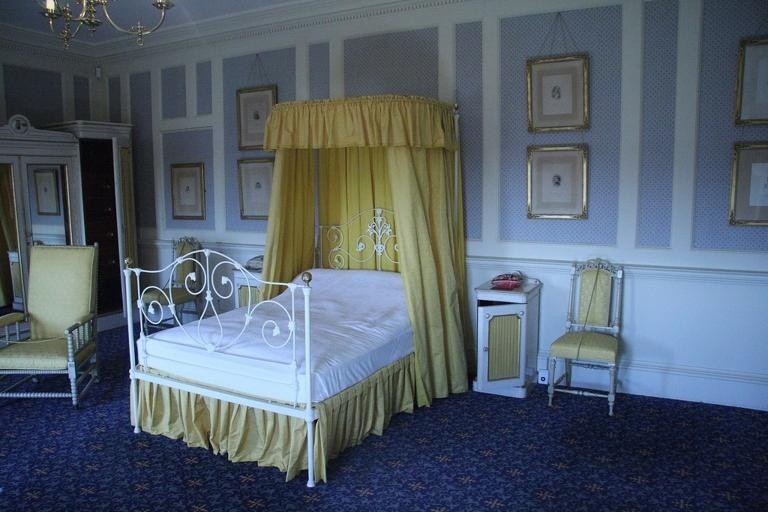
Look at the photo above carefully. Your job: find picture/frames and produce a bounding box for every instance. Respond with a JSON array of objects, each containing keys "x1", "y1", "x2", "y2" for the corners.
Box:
[
  {"x1": 728, "y1": 142, "x2": 768, "y2": 227},
  {"x1": 239, "y1": 157, "x2": 274, "y2": 220},
  {"x1": 171, "y1": 163, "x2": 204, "y2": 220},
  {"x1": 526, "y1": 143, "x2": 591, "y2": 220},
  {"x1": 734, "y1": 37, "x2": 768, "y2": 127},
  {"x1": 525, "y1": 52, "x2": 589, "y2": 131},
  {"x1": 33, "y1": 170, "x2": 61, "y2": 218},
  {"x1": 235, "y1": 86, "x2": 278, "y2": 151}
]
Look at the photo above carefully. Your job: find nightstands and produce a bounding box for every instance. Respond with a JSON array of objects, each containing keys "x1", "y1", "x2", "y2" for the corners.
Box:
[
  {"x1": 232, "y1": 266, "x2": 263, "y2": 309},
  {"x1": 472, "y1": 277, "x2": 542, "y2": 399}
]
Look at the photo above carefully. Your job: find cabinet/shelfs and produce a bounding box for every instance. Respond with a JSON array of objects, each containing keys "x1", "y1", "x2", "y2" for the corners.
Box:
[{"x1": 0, "y1": 116, "x2": 86, "y2": 343}]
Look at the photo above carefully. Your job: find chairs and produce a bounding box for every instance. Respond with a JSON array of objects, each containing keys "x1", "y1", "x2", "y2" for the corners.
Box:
[
  {"x1": 548, "y1": 259, "x2": 624, "y2": 414},
  {"x1": 140, "y1": 238, "x2": 202, "y2": 335},
  {"x1": 0, "y1": 243, "x2": 98, "y2": 406}
]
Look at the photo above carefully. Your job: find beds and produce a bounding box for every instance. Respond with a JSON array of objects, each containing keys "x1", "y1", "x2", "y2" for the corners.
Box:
[{"x1": 123, "y1": 208, "x2": 416, "y2": 486}]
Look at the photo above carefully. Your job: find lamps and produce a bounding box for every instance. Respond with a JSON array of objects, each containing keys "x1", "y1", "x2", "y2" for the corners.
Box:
[{"x1": 44, "y1": 1, "x2": 174, "y2": 46}]
